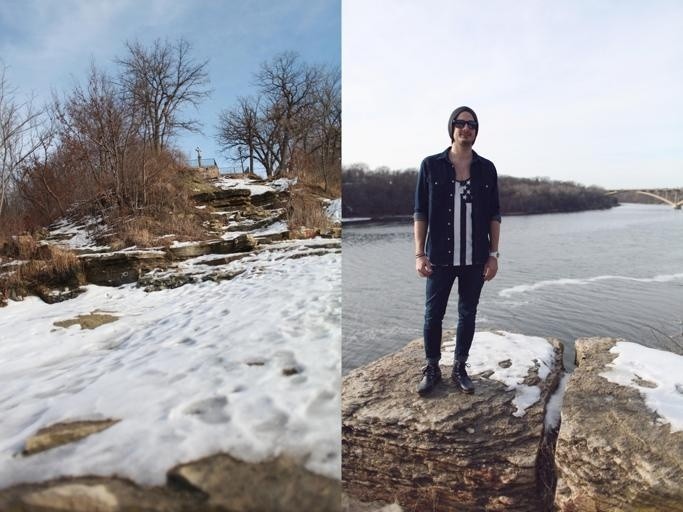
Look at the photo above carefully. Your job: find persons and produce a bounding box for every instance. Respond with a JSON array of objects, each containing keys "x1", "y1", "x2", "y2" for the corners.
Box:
[{"x1": 411, "y1": 106, "x2": 502, "y2": 396}]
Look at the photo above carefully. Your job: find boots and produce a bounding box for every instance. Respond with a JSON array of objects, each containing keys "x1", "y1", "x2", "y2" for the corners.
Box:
[
  {"x1": 415, "y1": 359, "x2": 441, "y2": 393},
  {"x1": 451, "y1": 358, "x2": 475, "y2": 394}
]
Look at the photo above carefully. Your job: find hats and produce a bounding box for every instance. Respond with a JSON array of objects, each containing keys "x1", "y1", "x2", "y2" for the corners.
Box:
[{"x1": 448, "y1": 106, "x2": 479, "y2": 139}]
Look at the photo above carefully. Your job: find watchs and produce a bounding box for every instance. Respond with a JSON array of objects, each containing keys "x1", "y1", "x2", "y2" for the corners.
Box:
[{"x1": 487, "y1": 250, "x2": 499, "y2": 260}]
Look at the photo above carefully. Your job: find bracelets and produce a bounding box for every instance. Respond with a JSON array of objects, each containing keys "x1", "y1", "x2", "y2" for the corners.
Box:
[{"x1": 414, "y1": 252, "x2": 425, "y2": 259}]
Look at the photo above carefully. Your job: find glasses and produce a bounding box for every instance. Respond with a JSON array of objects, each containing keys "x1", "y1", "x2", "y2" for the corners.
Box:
[{"x1": 453, "y1": 119, "x2": 478, "y2": 129}]
